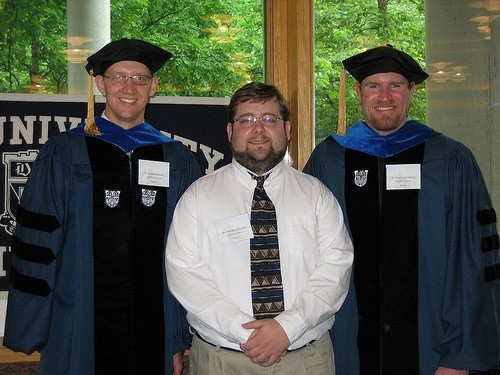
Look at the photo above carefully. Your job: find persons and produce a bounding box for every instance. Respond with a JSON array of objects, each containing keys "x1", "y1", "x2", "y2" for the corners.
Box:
[
  {"x1": 301, "y1": 44, "x2": 500, "y2": 375},
  {"x1": 164, "y1": 82, "x2": 354, "y2": 375},
  {"x1": 3, "y1": 38, "x2": 206, "y2": 375}
]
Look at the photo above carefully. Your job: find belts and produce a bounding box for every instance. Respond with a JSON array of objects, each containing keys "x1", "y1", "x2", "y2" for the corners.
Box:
[{"x1": 193, "y1": 330, "x2": 317, "y2": 354}]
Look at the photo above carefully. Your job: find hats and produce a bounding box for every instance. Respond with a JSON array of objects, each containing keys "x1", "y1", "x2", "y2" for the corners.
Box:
[
  {"x1": 343, "y1": 45, "x2": 429, "y2": 85},
  {"x1": 85, "y1": 38, "x2": 172, "y2": 78}
]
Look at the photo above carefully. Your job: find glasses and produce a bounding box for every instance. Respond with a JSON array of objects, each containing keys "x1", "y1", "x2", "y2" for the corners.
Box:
[
  {"x1": 102, "y1": 74, "x2": 154, "y2": 85},
  {"x1": 231, "y1": 114, "x2": 288, "y2": 126}
]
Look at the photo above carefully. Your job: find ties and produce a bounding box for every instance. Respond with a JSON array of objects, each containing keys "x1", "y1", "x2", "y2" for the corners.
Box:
[{"x1": 245, "y1": 171, "x2": 285, "y2": 319}]
